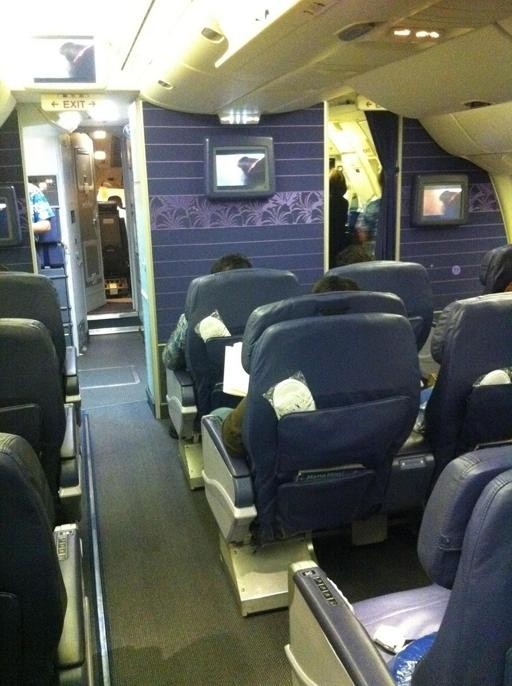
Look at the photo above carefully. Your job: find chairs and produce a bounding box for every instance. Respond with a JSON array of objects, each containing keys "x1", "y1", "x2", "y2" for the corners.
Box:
[
  {"x1": 202, "y1": 291, "x2": 420, "y2": 617},
  {"x1": 1, "y1": 272, "x2": 93, "y2": 686},
  {"x1": 286, "y1": 447, "x2": 512, "y2": 684},
  {"x1": 424, "y1": 291, "x2": 511, "y2": 447},
  {"x1": 166, "y1": 268, "x2": 304, "y2": 491},
  {"x1": 311, "y1": 260, "x2": 433, "y2": 354}
]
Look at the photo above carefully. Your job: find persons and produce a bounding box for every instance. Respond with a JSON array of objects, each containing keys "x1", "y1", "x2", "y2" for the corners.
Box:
[
  {"x1": 27, "y1": 182, "x2": 55, "y2": 273},
  {"x1": 329, "y1": 169, "x2": 348, "y2": 269},
  {"x1": 355, "y1": 172, "x2": 384, "y2": 241},
  {"x1": 220, "y1": 276, "x2": 361, "y2": 460},
  {"x1": 162, "y1": 252, "x2": 253, "y2": 372}
]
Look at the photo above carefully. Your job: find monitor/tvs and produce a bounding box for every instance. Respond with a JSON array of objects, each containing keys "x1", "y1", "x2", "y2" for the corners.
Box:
[
  {"x1": 0, "y1": 185, "x2": 22, "y2": 246},
  {"x1": 204, "y1": 134, "x2": 276, "y2": 199},
  {"x1": 412, "y1": 171, "x2": 469, "y2": 227}
]
